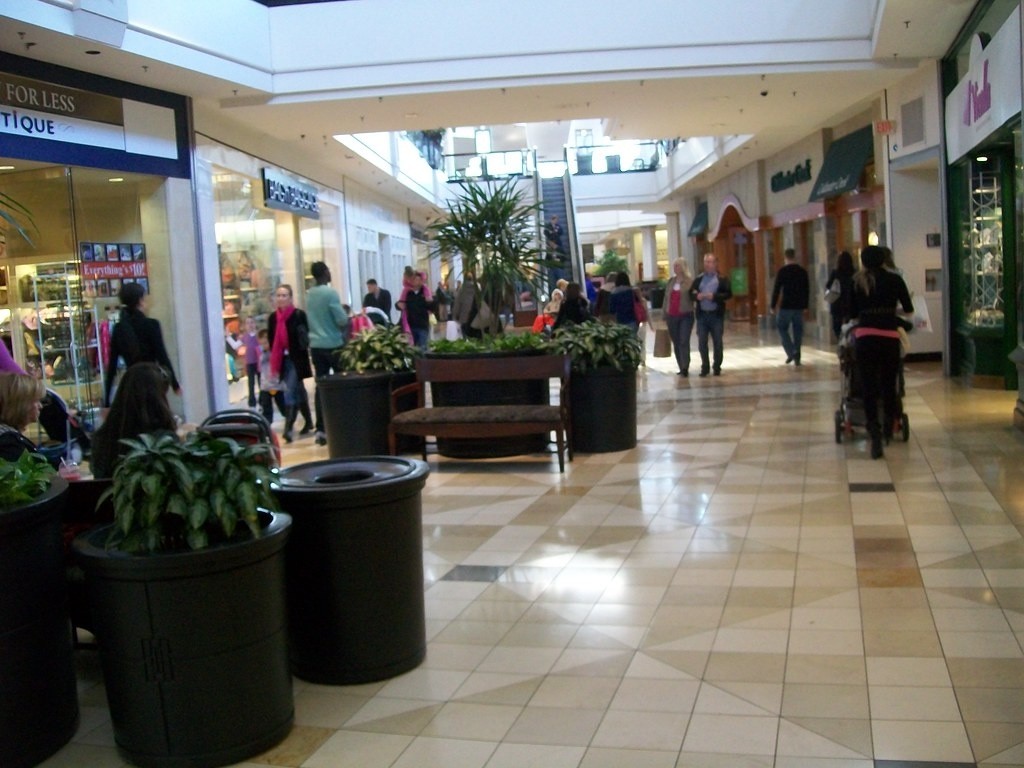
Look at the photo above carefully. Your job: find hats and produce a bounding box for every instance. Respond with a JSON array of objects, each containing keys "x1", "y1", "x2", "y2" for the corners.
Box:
[{"x1": 861, "y1": 245, "x2": 884, "y2": 269}]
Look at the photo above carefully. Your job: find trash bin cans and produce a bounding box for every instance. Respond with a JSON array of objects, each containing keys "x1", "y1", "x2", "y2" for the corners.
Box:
[{"x1": 270, "y1": 455, "x2": 429, "y2": 684}]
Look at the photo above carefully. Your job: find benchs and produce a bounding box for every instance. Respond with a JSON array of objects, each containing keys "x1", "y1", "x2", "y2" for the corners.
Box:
[{"x1": 389, "y1": 357, "x2": 573, "y2": 480}]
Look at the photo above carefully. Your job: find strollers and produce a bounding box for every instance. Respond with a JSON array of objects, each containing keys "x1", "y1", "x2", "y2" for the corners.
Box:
[
  {"x1": 833, "y1": 317, "x2": 909, "y2": 443},
  {"x1": 195, "y1": 410, "x2": 282, "y2": 470},
  {"x1": 33, "y1": 386, "x2": 93, "y2": 465}
]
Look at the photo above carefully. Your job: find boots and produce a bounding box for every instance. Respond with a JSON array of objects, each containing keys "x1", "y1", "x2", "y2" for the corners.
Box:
[
  {"x1": 884, "y1": 401, "x2": 895, "y2": 445},
  {"x1": 282, "y1": 401, "x2": 315, "y2": 443},
  {"x1": 863, "y1": 406, "x2": 883, "y2": 460}
]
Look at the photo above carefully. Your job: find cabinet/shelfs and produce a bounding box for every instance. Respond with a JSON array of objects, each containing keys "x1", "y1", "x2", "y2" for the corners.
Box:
[
  {"x1": 973, "y1": 187, "x2": 1001, "y2": 275},
  {"x1": 0, "y1": 249, "x2": 102, "y2": 404}
]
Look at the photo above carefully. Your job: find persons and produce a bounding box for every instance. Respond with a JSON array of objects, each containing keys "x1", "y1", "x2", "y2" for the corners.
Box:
[
  {"x1": 824, "y1": 246, "x2": 914, "y2": 459},
  {"x1": 689, "y1": 252, "x2": 732, "y2": 377},
  {"x1": 102, "y1": 283, "x2": 183, "y2": 420},
  {"x1": 662, "y1": 257, "x2": 697, "y2": 376},
  {"x1": 770, "y1": 248, "x2": 809, "y2": 366},
  {"x1": 225, "y1": 316, "x2": 288, "y2": 425},
  {"x1": 395, "y1": 266, "x2": 482, "y2": 349},
  {"x1": 267, "y1": 285, "x2": 313, "y2": 443},
  {"x1": 0, "y1": 337, "x2": 43, "y2": 462},
  {"x1": 91, "y1": 362, "x2": 196, "y2": 480},
  {"x1": 363, "y1": 279, "x2": 392, "y2": 317},
  {"x1": 543, "y1": 271, "x2": 654, "y2": 369},
  {"x1": 305, "y1": 262, "x2": 349, "y2": 446},
  {"x1": 544, "y1": 216, "x2": 565, "y2": 259}
]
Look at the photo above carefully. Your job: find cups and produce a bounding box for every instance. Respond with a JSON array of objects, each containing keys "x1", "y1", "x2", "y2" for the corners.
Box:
[{"x1": 60, "y1": 461, "x2": 81, "y2": 481}]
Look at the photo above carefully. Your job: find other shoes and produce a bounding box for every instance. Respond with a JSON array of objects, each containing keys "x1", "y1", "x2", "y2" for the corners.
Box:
[
  {"x1": 699, "y1": 370, "x2": 709, "y2": 377},
  {"x1": 713, "y1": 369, "x2": 720, "y2": 375},
  {"x1": 786, "y1": 349, "x2": 801, "y2": 366},
  {"x1": 677, "y1": 369, "x2": 688, "y2": 377}
]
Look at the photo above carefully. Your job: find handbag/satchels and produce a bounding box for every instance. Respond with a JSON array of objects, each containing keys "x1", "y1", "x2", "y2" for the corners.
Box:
[
  {"x1": 912, "y1": 293, "x2": 933, "y2": 334},
  {"x1": 633, "y1": 287, "x2": 646, "y2": 322},
  {"x1": 654, "y1": 329, "x2": 671, "y2": 357}
]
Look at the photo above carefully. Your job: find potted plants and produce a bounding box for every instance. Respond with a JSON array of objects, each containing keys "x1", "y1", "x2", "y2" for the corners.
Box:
[
  {"x1": 411, "y1": 170, "x2": 567, "y2": 458},
  {"x1": 552, "y1": 321, "x2": 645, "y2": 452},
  {"x1": 72, "y1": 433, "x2": 295, "y2": 768},
  {"x1": 376, "y1": 326, "x2": 428, "y2": 459},
  {"x1": 316, "y1": 324, "x2": 390, "y2": 462},
  {"x1": 0, "y1": 450, "x2": 80, "y2": 768}
]
